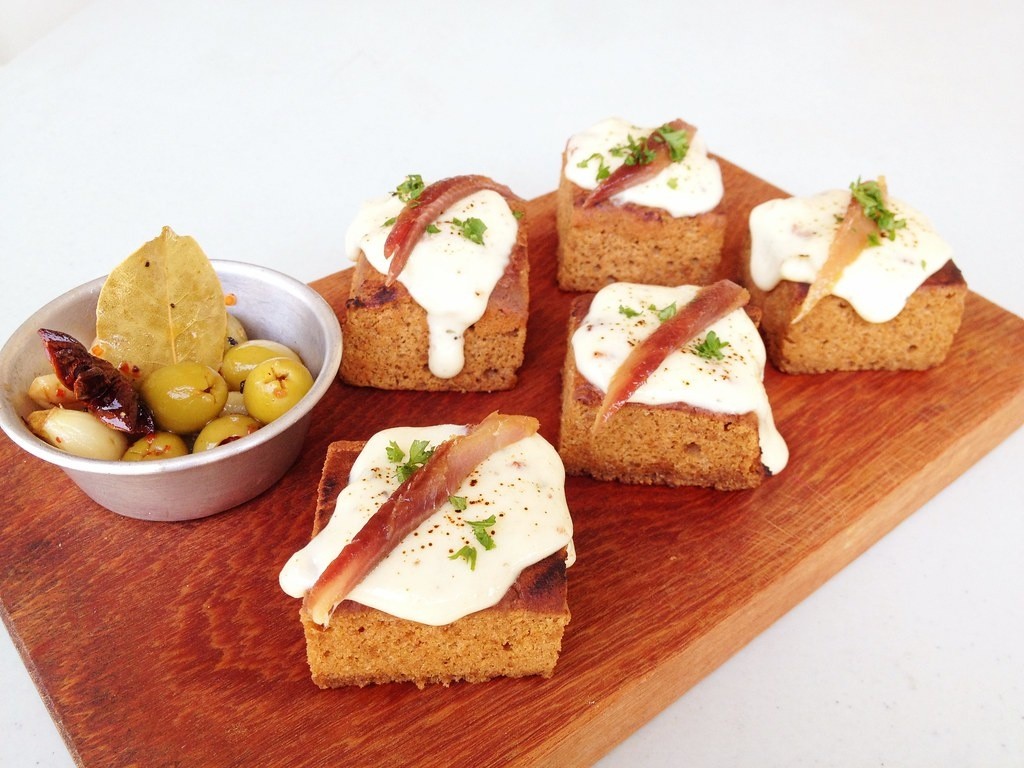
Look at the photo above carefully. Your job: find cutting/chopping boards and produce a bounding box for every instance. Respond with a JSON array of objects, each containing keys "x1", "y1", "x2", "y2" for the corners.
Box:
[{"x1": 3, "y1": 133, "x2": 1024, "y2": 767}]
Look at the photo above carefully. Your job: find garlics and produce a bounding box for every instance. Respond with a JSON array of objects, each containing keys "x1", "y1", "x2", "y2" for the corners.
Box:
[{"x1": 25, "y1": 373, "x2": 127, "y2": 467}]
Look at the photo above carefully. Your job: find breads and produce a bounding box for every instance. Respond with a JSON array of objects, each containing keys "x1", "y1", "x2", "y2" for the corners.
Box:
[{"x1": 278, "y1": 119, "x2": 971, "y2": 692}]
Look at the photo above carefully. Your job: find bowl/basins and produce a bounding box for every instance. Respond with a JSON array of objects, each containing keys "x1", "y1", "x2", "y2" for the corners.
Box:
[{"x1": 1, "y1": 255, "x2": 345, "y2": 523}]
